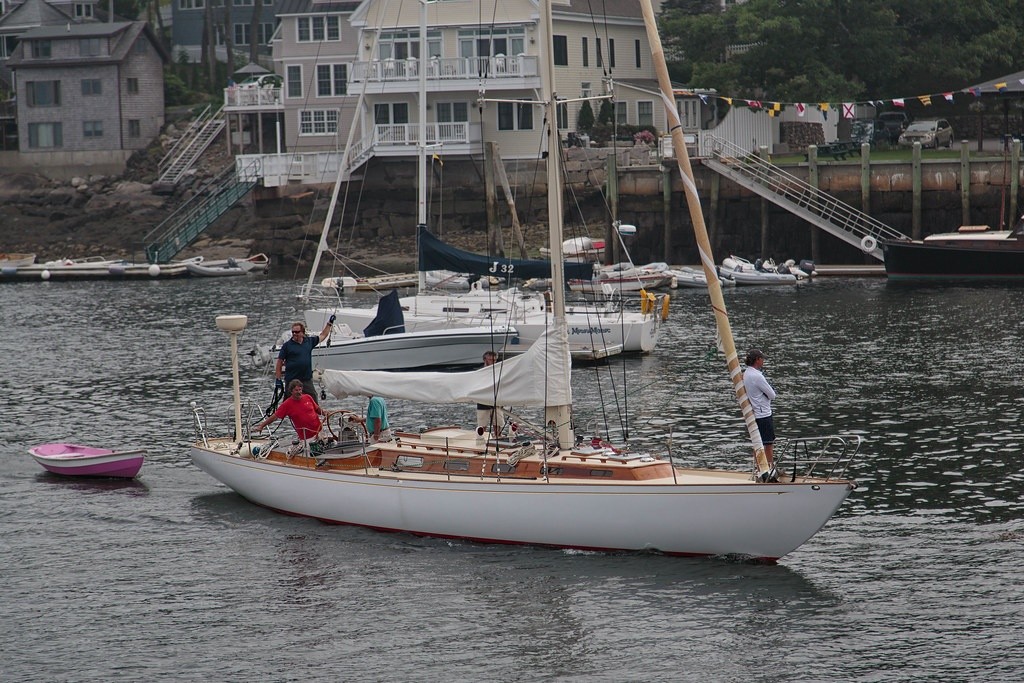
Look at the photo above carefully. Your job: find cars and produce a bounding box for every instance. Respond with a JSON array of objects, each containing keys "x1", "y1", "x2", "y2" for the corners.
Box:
[
  {"x1": 234, "y1": 74, "x2": 283, "y2": 104},
  {"x1": 850, "y1": 119, "x2": 893, "y2": 150}
]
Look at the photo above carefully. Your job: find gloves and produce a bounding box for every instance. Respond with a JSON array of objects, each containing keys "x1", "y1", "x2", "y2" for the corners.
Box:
[
  {"x1": 276, "y1": 378, "x2": 283, "y2": 388},
  {"x1": 327, "y1": 315, "x2": 336, "y2": 326}
]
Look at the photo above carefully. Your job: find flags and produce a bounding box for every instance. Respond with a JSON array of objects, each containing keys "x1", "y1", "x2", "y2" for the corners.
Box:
[
  {"x1": 748, "y1": 100, "x2": 762, "y2": 108},
  {"x1": 969, "y1": 87, "x2": 981, "y2": 97},
  {"x1": 944, "y1": 92, "x2": 953, "y2": 100},
  {"x1": 994, "y1": 83, "x2": 1007, "y2": 91},
  {"x1": 918, "y1": 95, "x2": 932, "y2": 107},
  {"x1": 720, "y1": 96, "x2": 734, "y2": 106},
  {"x1": 817, "y1": 103, "x2": 830, "y2": 121},
  {"x1": 842, "y1": 103, "x2": 855, "y2": 118},
  {"x1": 795, "y1": 103, "x2": 806, "y2": 117},
  {"x1": 767, "y1": 102, "x2": 780, "y2": 117},
  {"x1": 698, "y1": 93, "x2": 710, "y2": 105},
  {"x1": 433, "y1": 154, "x2": 443, "y2": 167},
  {"x1": 892, "y1": 98, "x2": 904, "y2": 107}
]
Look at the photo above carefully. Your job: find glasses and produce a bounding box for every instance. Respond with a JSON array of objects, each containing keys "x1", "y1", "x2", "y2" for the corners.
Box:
[{"x1": 292, "y1": 331, "x2": 304, "y2": 334}]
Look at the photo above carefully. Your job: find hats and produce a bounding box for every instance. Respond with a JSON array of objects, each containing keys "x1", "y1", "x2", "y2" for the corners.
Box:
[{"x1": 747, "y1": 349, "x2": 766, "y2": 359}]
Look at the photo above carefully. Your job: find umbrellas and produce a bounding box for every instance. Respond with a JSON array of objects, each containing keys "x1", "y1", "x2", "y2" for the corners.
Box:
[{"x1": 234, "y1": 62, "x2": 271, "y2": 81}]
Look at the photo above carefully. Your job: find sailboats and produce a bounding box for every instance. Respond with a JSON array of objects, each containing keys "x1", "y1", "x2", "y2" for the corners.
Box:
[
  {"x1": 304, "y1": 0, "x2": 662, "y2": 352},
  {"x1": 192, "y1": 0, "x2": 855, "y2": 564}
]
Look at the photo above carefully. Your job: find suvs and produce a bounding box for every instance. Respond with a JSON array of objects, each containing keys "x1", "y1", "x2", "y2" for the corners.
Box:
[
  {"x1": 872, "y1": 111, "x2": 910, "y2": 145},
  {"x1": 898, "y1": 118, "x2": 955, "y2": 150}
]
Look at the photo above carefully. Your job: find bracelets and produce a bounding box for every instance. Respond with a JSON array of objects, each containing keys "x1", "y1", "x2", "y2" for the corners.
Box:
[{"x1": 375, "y1": 435, "x2": 379, "y2": 436}]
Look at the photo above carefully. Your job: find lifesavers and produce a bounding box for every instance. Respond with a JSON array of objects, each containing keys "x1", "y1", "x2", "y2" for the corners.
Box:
[
  {"x1": 662, "y1": 294, "x2": 670, "y2": 323},
  {"x1": 861, "y1": 235, "x2": 878, "y2": 252}
]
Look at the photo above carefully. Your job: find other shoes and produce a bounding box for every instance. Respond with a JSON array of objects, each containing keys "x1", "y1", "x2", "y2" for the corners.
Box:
[{"x1": 776, "y1": 468, "x2": 785, "y2": 477}]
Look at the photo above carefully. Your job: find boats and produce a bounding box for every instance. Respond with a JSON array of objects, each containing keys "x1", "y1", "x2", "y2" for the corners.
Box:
[
  {"x1": 302, "y1": 276, "x2": 357, "y2": 295},
  {"x1": 355, "y1": 273, "x2": 419, "y2": 288},
  {"x1": 719, "y1": 255, "x2": 818, "y2": 285},
  {"x1": 881, "y1": 215, "x2": 1024, "y2": 286},
  {"x1": 0, "y1": 255, "x2": 204, "y2": 280},
  {"x1": 271, "y1": 325, "x2": 519, "y2": 374},
  {"x1": 426, "y1": 270, "x2": 468, "y2": 290},
  {"x1": 0, "y1": 252, "x2": 36, "y2": 266},
  {"x1": 185, "y1": 259, "x2": 254, "y2": 275},
  {"x1": 665, "y1": 267, "x2": 724, "y2": 289},
  {"x1": 538, "y1": 238, "x2": 608, "y2": 262},
  {"x1": 28, "y1": 443, "x2": 148, "y2": 479},
  {"x1": 568, "y1": 270, "x2": 668, "y2": 295},
  {"x1": 682, "y1": 266, "x2": 737, "y2": 288}
]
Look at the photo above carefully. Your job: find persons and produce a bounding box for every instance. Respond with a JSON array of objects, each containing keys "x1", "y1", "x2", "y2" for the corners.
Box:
[
  {"x1": 353, "y1": 395, "x2": 392, "y2": 446},
  {"x1": 254, "y1": 379, "x2": 331, "y2": 447},
  {"x1": 743, "y1": 348, "x2": 786, "y2": 477},
  {"x1": 276, "y1": 315, "x2": 336, "y2": 406},
  {"x1": 227, "y1": 74, "x2": 234, "y2": 87},
  {"x1": 476, "y1": 351, "x2": 505, "y2": 433}
]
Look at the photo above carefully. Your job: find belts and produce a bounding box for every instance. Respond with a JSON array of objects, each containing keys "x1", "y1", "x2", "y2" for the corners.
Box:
[{"x1": 370, "y1": 428, "x2": 389, "y2": 436}]
